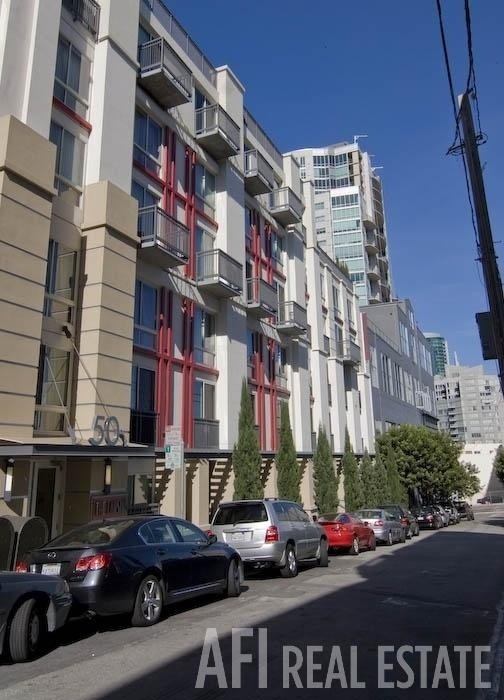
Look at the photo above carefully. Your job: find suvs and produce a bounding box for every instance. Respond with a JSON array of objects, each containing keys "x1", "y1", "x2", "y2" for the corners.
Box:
[{"x1": 205, "y1": 498, "x2": 330, "y2": 577}]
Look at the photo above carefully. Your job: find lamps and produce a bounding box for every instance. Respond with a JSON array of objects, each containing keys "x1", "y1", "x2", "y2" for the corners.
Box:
[
  {"x1": 4, "y1": 458, "x2": 15, "y2": 502},
  {"x1": 105, "y1": 457, "x2": 113, "y2": 494}
]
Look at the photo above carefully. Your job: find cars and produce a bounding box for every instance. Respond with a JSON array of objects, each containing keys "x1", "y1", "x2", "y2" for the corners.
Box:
[
  {"x1": 316, "y1": 514, "x2": 376, "y2": 555},
  {"x1": 356, "y1": 498, "x2": 475, "y2": 546},
  {"x1": 0, "y1": 570, "x2": 73, "y2": 665},
  {"x1": 16, "y1": 514, "x2": 246, "y2": 626}
]
